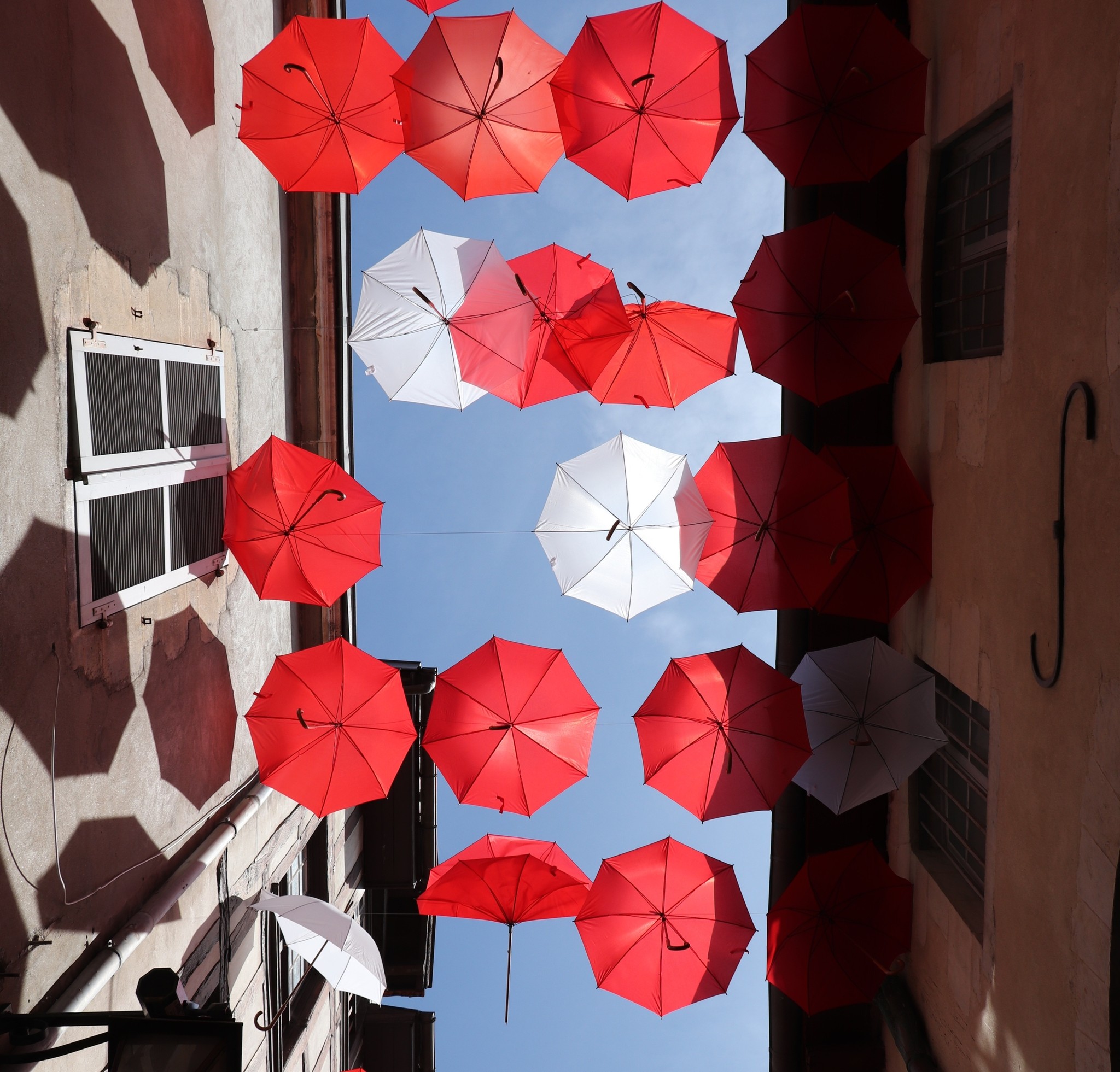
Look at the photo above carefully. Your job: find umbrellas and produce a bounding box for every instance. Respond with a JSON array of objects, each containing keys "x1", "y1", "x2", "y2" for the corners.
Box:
[
  {"x1": 339, "y1": 226, "x2": 538, "y2": 412},
  {"x1": 807, "y1": 441, "x2": 935, "y2": 625},
  {"x1": 730, "y1": 217, "x2": 924, "y2": 408},
  {"x1": 422, "y1": 635, "x2": 604, "y2": 819},
  {"x1": 219, "y1": 432, "x2": 387, "y2": 610},
  {"x1": 573, "y1": 836, "x2": 757, "y2": 1020},
  {"x1": 740, "y1": 6, "x2": 932, "y2": 190},
  {"x1": 762, "y1": 841, "x2": 916, "y2": 1019},
  {"x1": 447, "y1": 244, "x2": 635, "y2": 412},
  {"x1": 631, "y1": 644, "x2": 813, "y2": 827},
  {"x1": 674, "y1": 433, "x2": 861, "y2": 616},
  {"x1": 546, "y1": 2, "x2": 741, "y2": 203},
  {"x1": 243, "y1": 636, "x2": 418, "y2": 819},
  {"x1": 250, "y1": 894, "x2": 388, "y2": 1033},
  {"x1": 579, "y1": 281, "x2": 740, "y2": 413},
  {"x1": 782, "y1": 637, "x2": 948, "y2": 818},
  {"x1": 411, "y1": 832, "x2": 594, "y2": 1026},
  {"x1": 231, "y1": 14, "x2": 407, "y2": 196},
  {"x1": 387, "y1": 8, "x2": 568, "y2": 204}
]
